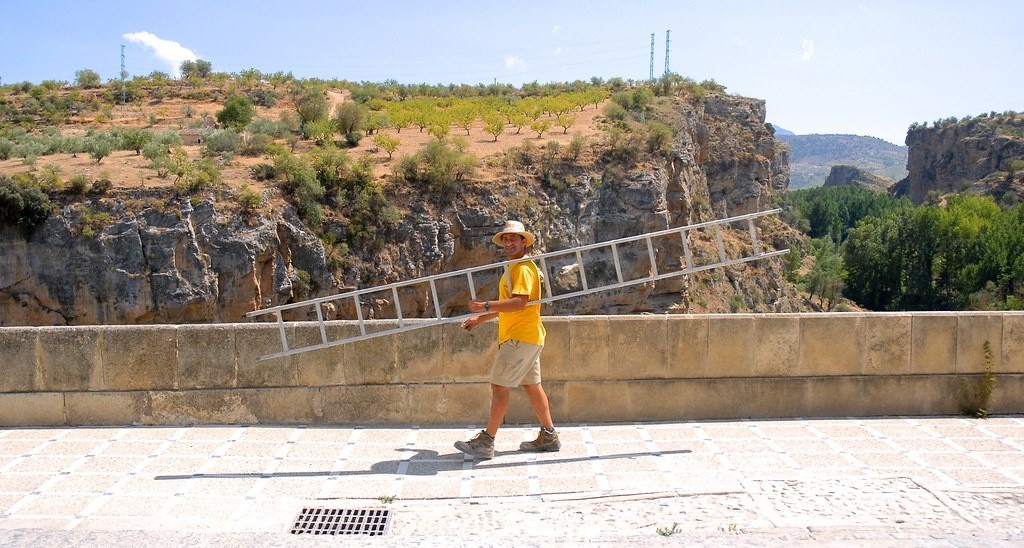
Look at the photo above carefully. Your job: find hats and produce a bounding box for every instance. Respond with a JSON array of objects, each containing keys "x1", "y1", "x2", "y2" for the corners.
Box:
[{"x1": 491, "y1": 220, "x2": 535, "y2": 248}]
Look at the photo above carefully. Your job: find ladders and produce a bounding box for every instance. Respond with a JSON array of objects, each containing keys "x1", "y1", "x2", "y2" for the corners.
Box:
[{"x1": 245, "y1": 207, "x2": 791, "y2": 364}]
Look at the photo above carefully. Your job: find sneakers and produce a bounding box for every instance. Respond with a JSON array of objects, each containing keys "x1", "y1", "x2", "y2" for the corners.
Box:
[
  {"x1": 454, "y1": 430, "x2": 495, "y2": 460},
  {"x1": 520, "y1": 426, "x2": 560, "y2": 452}
]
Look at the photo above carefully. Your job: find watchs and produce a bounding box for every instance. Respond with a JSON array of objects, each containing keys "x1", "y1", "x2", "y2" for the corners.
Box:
[{"x1": 484, "y1": 301, "x2": 490, "y2": 311}]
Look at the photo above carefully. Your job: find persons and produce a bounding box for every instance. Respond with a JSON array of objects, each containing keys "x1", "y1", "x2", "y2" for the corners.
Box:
[{"x1": 454, "y1": 220, "x2": 561, "y2": 458}]
